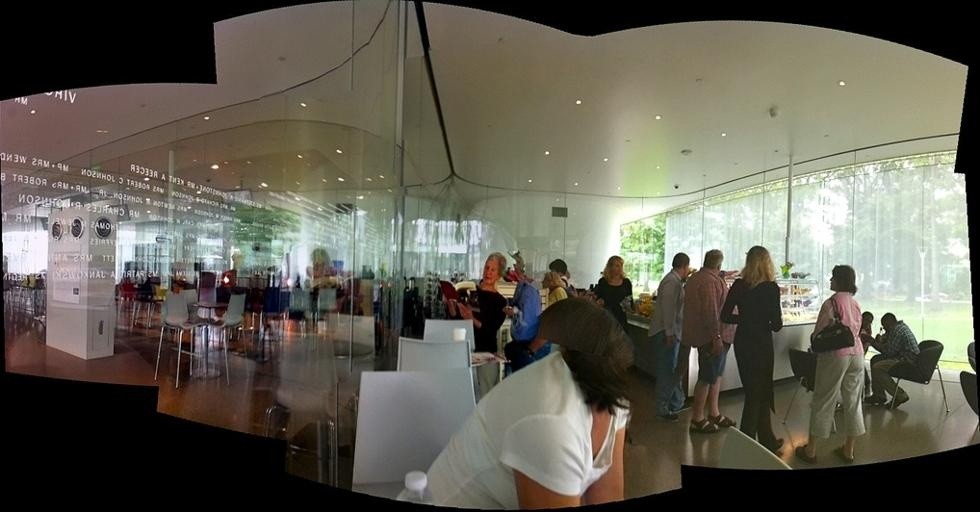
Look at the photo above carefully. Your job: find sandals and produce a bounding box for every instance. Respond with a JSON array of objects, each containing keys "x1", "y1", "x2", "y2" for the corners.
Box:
[
  {"x1": 708, "y1": 414, "x2": 737, "y2": 426},
  {"x1": 689, "y1": 417, "x2": 720, "y2": 433}
]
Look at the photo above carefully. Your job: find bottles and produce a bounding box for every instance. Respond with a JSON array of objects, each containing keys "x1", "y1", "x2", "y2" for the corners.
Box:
[
  {"x1": 879, "y1": 327, "x2": 883, "y2": 336},
  {"x1": 636, "y1": 293, "x2": 653, "y2": 317},
  {"x1": 395, "y1": 469, "x2": 434, "y2": 504}
]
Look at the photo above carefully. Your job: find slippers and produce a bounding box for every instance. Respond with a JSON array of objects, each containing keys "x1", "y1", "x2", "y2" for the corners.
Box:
[
  {"x1": 834, "y1": 446, "x2": 853, "y2": 464},
  {"x1": 796, "y1": 447, "x2": 816, "y2": 464}
]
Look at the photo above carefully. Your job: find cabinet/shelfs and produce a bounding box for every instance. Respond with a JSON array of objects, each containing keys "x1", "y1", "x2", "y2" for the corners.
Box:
[{"x1": 774, "y1": 276, "x2": 821, "y2": 326}]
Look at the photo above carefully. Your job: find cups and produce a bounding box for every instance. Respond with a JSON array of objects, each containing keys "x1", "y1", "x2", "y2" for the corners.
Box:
[
  {"x1": 453, "y1": 328, "x2": 466, "y2": 341},
  {"x1": 317, "y1": 317, "x2": 327, "y2": 333}
]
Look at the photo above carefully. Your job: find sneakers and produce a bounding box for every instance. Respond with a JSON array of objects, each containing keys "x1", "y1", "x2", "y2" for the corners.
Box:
[
  {"x1": 671, "y1": 404, "x2": 691, "y2": 413},
  {"x1": 887, "y1": 393, "x2": 909, "y2": 409},
  {"x1": 865, "y1": 394, "x2": 887, "y2": 403},
  {"x1": 656, "y1": 413, "x2": 682, "y2": 422}
]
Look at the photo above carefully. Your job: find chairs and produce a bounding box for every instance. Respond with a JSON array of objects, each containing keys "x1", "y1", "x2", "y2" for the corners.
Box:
[
  {"x1": 1, "y1": 268, "x2": 556, "y2": 506},
  {"x1": 959, "y1": 370, "x2": 980, "y2": 443},
  {"x1": 780, "y1": 346, "x2": 838, "y2": 433},
  {"x1": 887, "y1": 339, "x2": 950, "y2": 413}
]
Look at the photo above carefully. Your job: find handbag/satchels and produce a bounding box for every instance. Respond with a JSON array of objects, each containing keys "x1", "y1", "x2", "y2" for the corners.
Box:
[{"x1": 810, "y1": 323, "x2": 855, "y2": 352}]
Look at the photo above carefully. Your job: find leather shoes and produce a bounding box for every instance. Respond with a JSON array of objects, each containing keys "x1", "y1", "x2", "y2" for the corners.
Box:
[{"x1": 775, "y1": 439, "x2": 784, "y2": 449}]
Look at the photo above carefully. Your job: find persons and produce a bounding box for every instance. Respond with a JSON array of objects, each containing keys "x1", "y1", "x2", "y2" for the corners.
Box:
[
  {"x1": 395, "y1": 302, "x2": 635, "y2": 511},
  {"x1": 116, "y1": 244, "x2": 924, "y2": 465}
]
